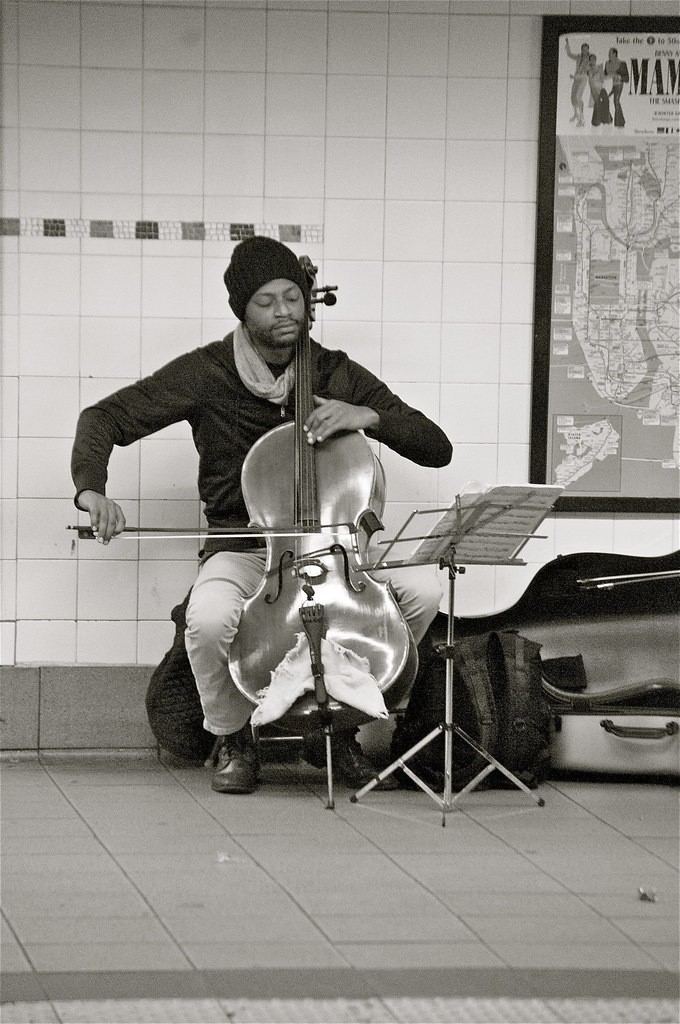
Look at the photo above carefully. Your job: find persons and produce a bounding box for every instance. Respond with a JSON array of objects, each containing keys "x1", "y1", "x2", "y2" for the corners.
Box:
[{"x1": 71, "y1": 236, "x2": 453, "y2": 794}]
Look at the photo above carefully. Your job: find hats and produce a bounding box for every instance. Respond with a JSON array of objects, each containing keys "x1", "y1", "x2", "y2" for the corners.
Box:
[{"x1": 224, "y1": 236, "x2": 309, "y2": 323}]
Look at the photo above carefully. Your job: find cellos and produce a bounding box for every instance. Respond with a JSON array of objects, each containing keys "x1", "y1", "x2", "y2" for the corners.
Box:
[{"x1": 226, "y1": 255, "x2": 422, "y2": 734}]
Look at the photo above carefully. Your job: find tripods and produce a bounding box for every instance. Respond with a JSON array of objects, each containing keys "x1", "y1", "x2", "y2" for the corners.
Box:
[{"x1": 349, "y1": 484, "x2": 566, "y2": 829}]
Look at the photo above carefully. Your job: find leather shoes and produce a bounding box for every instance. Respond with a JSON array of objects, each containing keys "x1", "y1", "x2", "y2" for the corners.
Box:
[
  {"x1": 300, "y1": 728, "x2": 399, "y2": 790},
  {"x1": 210, "y1": 714, "x2": 260, "y2": 793}
]
